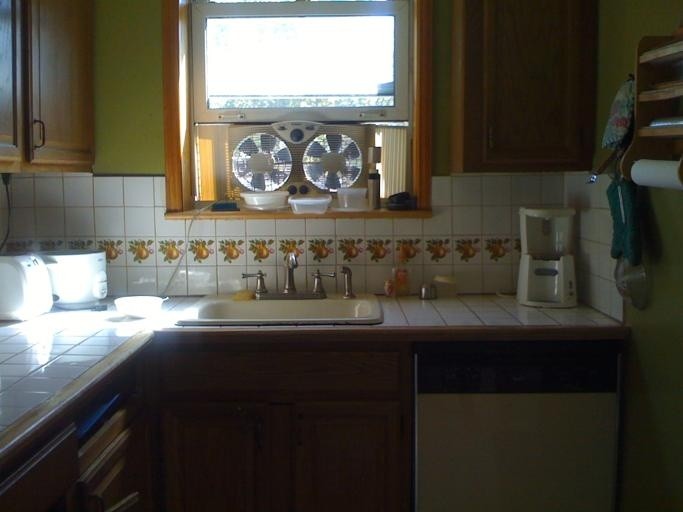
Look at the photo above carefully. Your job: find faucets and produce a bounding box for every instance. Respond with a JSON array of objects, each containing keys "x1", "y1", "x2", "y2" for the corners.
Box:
[
  {"x1": 339, "y1": 265, "x2": 352, "y2": 295},
  {"x1": 282, "y1": 252, "x2": 299, "y2": 294}
]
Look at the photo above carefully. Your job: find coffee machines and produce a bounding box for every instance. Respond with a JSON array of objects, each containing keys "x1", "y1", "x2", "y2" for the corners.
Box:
[{"x1": 517, "y1": 207, "x2": 577, "y2": 308}]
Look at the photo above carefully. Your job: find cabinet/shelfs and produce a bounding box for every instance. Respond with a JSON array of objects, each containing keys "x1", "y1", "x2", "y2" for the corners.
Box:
[
  {"x1": 475, "y1": 2, "x2": 587, "y2": 170},
  {"x1": 144, "y1": 344, "x2": 412, "y2": 511},
  {"x1": 633, "y1": 35, "x2": 683, "y2": 138},
  {"x1": 0, "y1": 0, "x2": 94, "y2": 167},
  {"x1": 0, "y1": 358, "x2": 154, "y2": 511}
]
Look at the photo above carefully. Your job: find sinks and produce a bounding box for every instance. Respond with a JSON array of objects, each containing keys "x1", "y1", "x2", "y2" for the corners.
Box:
[{"x1": 174, "y1": 294, "x2": 385, "y2": 325}]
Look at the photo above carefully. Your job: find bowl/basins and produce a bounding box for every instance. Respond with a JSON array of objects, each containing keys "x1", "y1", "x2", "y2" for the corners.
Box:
[
  {"x1": 241, "y1": 192, "x2": 290, "y2": 207},
  {"x1": 114, "y1": 296, "x2": 162, "y2": 317}
]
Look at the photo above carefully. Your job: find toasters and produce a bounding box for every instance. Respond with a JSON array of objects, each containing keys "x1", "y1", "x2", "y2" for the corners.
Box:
[{"x1": 0, "y1": 251, "x2": 53, "y2": 321}]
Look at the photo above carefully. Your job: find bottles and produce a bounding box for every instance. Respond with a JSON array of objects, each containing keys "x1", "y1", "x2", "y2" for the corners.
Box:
[{"x1": 368, "y1": 170, "x2": 381, "y2": 212}]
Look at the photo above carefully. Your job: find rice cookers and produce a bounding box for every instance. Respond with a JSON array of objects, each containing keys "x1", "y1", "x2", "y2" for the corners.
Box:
[{"x1": 38, "y1": 251, "x2": 106, "y2": 310}]
